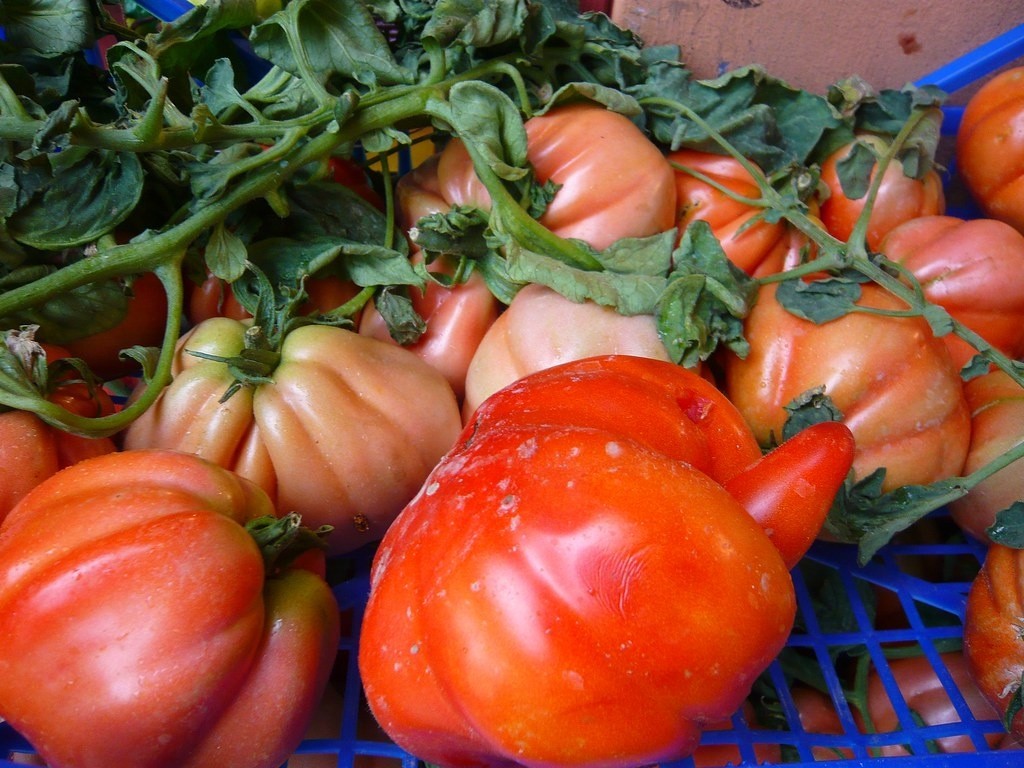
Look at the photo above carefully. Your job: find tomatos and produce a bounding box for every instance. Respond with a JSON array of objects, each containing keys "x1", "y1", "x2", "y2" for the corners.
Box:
[{"x1": 1, "y1": 63, "x2": 1024, "y2": 768}]
